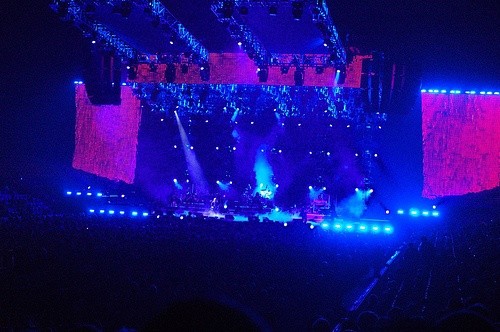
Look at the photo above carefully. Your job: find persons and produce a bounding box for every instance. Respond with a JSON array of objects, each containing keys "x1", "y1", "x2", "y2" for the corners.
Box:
[
  {"x1": 299, "y1": 201, "x2": 311, "y2": 223},
  {"x1": 167, "y1": 194, "x2": 206, "y2": 209},
  {"x1": 211, "y1": 198, "x2": 218, "y2": 213}
]
